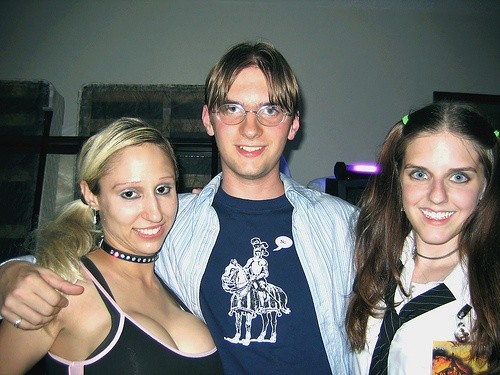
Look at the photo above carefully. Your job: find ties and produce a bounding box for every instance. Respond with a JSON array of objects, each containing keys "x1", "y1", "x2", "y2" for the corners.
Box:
[{"x1": 368, "y1": 259, "x2": 456, "y2": 375}]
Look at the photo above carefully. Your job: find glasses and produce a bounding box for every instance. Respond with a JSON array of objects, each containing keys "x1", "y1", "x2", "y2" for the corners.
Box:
[{"x1": 208, "y1": 103, "x2": 297, "y2": 127}]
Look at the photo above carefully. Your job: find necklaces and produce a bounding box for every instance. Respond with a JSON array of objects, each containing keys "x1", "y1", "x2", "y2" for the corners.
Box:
[
  {"x1": 415, "y1": 245, "x2": 461, "y2": 260},
  {"x1": 93, "y1": 235, "x2": 159, "y2": 264}
]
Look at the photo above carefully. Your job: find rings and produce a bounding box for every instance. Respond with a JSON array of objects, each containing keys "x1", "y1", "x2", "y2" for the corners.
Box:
[{"x1": 13, "y1": 317, "x2": 24, "y2": 329}]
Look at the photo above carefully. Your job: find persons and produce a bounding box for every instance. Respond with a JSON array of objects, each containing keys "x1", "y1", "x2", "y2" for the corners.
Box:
[
  {"x1": 345, "y1": 101, "x2": 500, "y2": 375},
  {"x1": 0, "y1": 41, "x2": 363, "y2": 375},
  {"x1": 0, "y1": 116, "x2": 226, "y2": 375}
]
